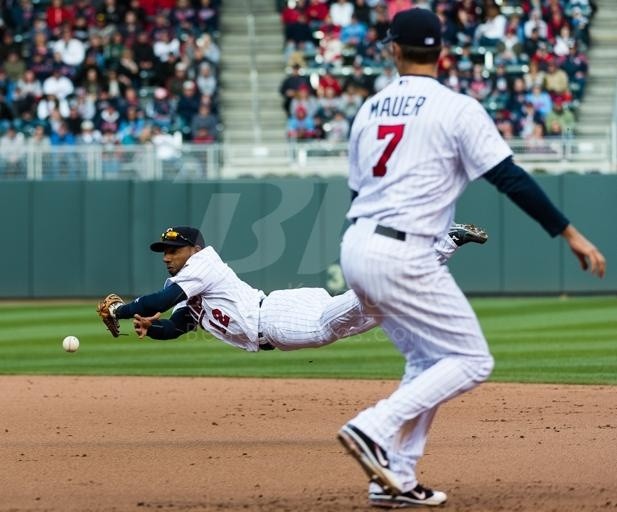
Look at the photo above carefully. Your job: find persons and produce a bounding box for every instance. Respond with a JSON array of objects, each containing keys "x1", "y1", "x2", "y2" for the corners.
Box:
[
  {"x1": 94, "y1": 218, "x2": 489, "y2": 353},
  {"x1": 335, "y1": 8, "x2": 609, "y2": 511},
  {"x1": 274, "y1": 1, "x2": 595, "y2": 156},
  {"x1": 0, "y1": 1, "x2": 226, "y2": 180}
]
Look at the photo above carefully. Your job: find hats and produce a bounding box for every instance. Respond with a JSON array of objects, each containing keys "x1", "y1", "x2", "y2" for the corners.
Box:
[
  {"x1": 149, "y1": 226, "x2": 204, "y2": 252},
  {"x1": 380, "y1": 8, "x2": 440, "y2": 48}
]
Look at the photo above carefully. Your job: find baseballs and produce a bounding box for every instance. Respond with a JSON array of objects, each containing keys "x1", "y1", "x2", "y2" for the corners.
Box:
[{"x1": 63, "y1": 336, "x2": 80, "y2": 352}]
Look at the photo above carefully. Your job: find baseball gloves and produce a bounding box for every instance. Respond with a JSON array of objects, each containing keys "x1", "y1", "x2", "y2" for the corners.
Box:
[{"x1": 98, "y1": 294, "x2": 127, "y2": 336}]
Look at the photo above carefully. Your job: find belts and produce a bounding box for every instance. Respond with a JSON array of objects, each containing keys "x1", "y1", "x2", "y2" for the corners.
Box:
[
  {"x1": 257, "y1": 299, "x2": 274, "y2": 351},
  {"x1": 352, "y1": 218, "x2": 405, "y2": 242}
]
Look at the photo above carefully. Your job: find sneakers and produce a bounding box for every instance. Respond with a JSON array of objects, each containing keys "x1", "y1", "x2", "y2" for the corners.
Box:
[
  {"x1": 367, "y1": 479, "x2": 448, "y2": 509},
  {"x1": 336, "y1": 423, "x2": 404, "y2": 497},
  {"x1": 447, "y1": 222, "x2": 488, "y2": 247}
]
